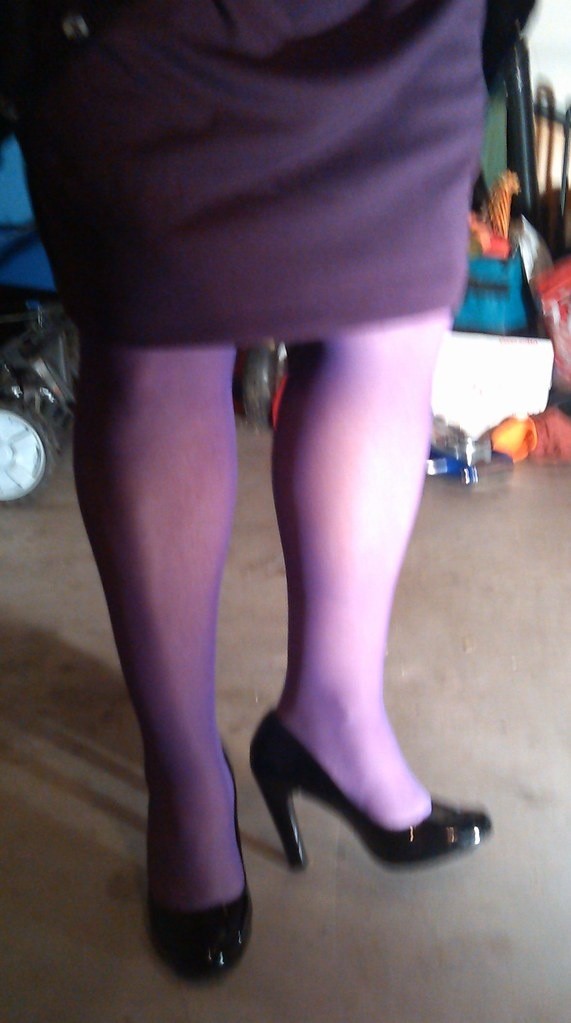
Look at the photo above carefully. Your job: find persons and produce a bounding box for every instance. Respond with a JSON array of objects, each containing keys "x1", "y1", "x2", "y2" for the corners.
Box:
[{"x1": 0, "y1": 1, "x2": 492, "y2": 985}]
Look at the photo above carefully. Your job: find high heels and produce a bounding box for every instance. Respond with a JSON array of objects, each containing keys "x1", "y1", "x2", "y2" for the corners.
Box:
[
  {"x1": 247, "y1": 708, "x2": 495, "y2": 870},
  {"x1": 144, "y1": 742, "x2": 252, "y2": 982}
]
